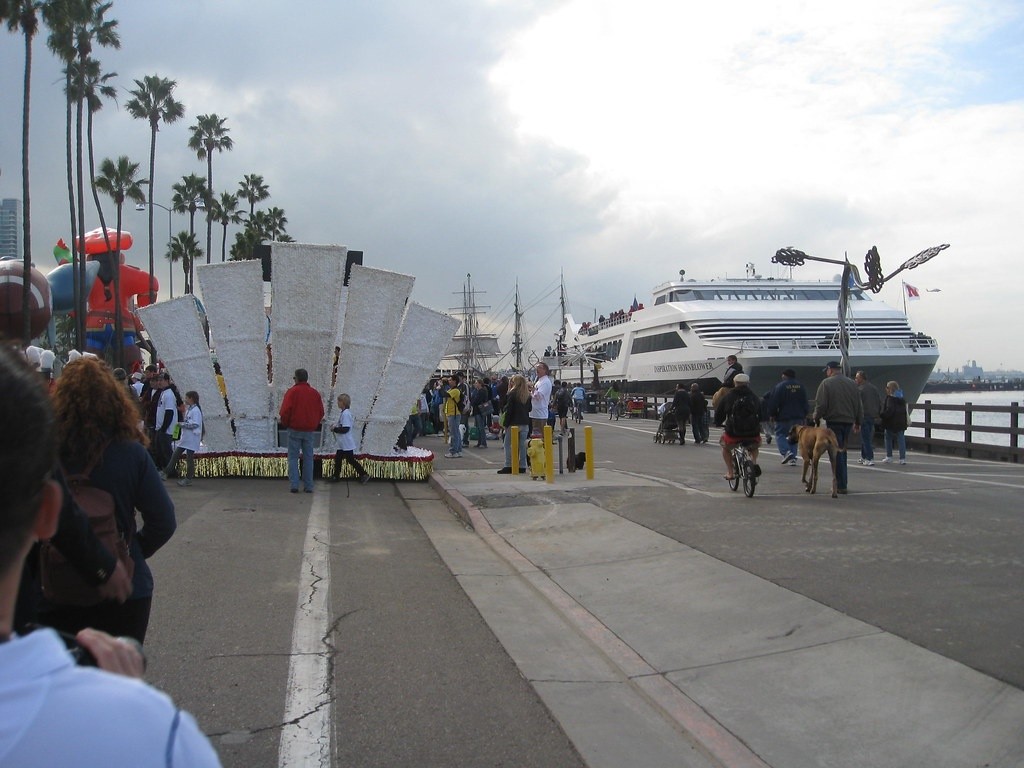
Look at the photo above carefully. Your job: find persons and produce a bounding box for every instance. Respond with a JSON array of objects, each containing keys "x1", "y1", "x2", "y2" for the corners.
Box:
[
  {"x1": 879, "y1": 381, "x2": 908, "y2": 464},
  {"x1": 279, "y1": 368, "x2": 324, "y2": 493},
  {"x1": 714, "y1": 373, "x2": 761, "y2": 480},
  {"x1": 0, "y1": 337, "x2": 226, "y2": 768},
  {"x1": 406, "y1": 362, "x2": 585, "y2": 474},
  {"x1": 604, "y1": 382, "x2": 620, "y2": 418},
  {"x1": 670, "y1": 355, "x2": 776, "y2": 445},
  {"x1": 769, "y1": 369, "x2": 808, "y2": 465},
  {"x1": 326, "y1": 393, "x2": 370, "y2": 485},
  {"x1": 853, "y1": 370, "x2": 882, "y2": 466},
  {"x1": 813, "y1": 362, "x2": 866, "y2": 494}
]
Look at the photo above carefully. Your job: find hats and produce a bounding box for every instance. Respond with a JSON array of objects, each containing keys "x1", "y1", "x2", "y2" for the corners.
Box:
[
  {"x1": 821, "y1": 361, "x2": 842, "y2": 372},
  {"x1": 733, "y1": 373, "x2": 751, "y2": 385},
  {"x1": 153, "y1": 372, "x2": 170, "y2": 380},
  {"x1": 130, "y1": 371, "x2": 142, "y2": 380},
  {"x1": 782, "y1": 368, "x2": 796, "y2": 379},
  {"x1": 22, "y1": 346, "x2": 98, "y2": 373},
  {"x1": 112, "y1": 368, "x2": 129, "y2": 381}
]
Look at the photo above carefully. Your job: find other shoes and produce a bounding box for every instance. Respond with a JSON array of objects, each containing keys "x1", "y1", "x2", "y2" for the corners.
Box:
[
  {"x1": 361, "y1": 474, "x2": 370, "y2": 485},
  {"x1": 519, "y1": 468, "x2": 527, "y2": 474},
  {"x1": 158, "y1": 470, "x2": 168, "y2": 482},
  {"x1": 291, "y1": 488, "x2": 298, "y2": 493},
  {"x1": 304, "y1": 488, "x2": 312, "y2": 493},
  {"x1": 497, "y1": 466, "x2": 512, "y2": 474},
  {"x1": 400, "y1": 425, "x2": 573, "y2": 465},
  {"x1": 325, "y1": 475, "x2": 340, "y2": 482},
  {"x1": 176, "y1": 478, "x2": 193, "y2": 486}
]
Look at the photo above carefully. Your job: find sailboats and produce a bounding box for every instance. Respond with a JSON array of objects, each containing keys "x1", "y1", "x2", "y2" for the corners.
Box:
[{"x1": 426, "y1": 272, "x2": 512, "y2": 381}]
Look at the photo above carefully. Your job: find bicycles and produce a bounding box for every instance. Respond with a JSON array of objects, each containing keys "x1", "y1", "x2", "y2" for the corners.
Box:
[
  {"x1": 604, "y1": 396, "x2": 619, "y2": 421},
  {"x1": 575, "y1": 401, "x2": 582, "y2": 424},
  {"x1": 718, "y1": 424, "x2": 757, "y2": 498}
]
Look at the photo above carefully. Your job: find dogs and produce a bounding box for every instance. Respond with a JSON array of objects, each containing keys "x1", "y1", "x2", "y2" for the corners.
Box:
[
  {"x1": 786, "y1": 425, "x2": 847, "y2": 498},
  {"x1": 567, "y1": 452, "x2": 586, "y2": 470}
]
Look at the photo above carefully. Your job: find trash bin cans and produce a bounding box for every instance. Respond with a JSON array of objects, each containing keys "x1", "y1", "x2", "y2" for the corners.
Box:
[{"x1": 584, "y1": 391, "x2": 598, "y2": 414}]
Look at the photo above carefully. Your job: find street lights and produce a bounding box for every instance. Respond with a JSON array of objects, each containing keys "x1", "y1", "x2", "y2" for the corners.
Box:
[{"x1": 136, "y1": 198, "x2": 204, "y2": 300}]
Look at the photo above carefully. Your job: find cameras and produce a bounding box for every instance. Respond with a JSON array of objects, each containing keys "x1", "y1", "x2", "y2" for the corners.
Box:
[{"x1": 22, "y1": 623, "x2": 147, "y2": 675}]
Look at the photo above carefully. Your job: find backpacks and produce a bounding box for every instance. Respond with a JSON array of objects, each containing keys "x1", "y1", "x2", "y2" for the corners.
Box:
[
  {"x1": 727, "y1": 388, "x2": 762, "y2": 437},
  {"x1": 40, "y1": 434, "x2": 136, "y2": 601}
]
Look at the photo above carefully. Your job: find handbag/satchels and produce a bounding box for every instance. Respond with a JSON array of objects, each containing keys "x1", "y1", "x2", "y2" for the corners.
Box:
[
  {"x1": 172, "y1": 422, "x2": 180, "y2": 439},
  {"x1": 476, "y1": 389, "x2": 494, "y2": 417},
  {"x1": 451, "y1": 387, "x2": 471, "y2": 414},
  {"x1": 499, "y1": 411, "x2": 506, "y2": 429}
]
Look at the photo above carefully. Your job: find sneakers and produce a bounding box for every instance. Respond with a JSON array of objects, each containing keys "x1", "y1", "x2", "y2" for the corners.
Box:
[{"x1": 680, "y1": 430, "x2": 908, "y2": 495}]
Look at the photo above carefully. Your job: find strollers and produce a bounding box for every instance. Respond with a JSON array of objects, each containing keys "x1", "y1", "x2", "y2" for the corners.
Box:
[{"x1": 653, "y1": 402, "x2": 680, "y2": 444}]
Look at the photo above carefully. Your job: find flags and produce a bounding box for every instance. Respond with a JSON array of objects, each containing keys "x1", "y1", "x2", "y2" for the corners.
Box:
[{"x1": 904, "y1": 282, "x2": 922, "y2": 300}]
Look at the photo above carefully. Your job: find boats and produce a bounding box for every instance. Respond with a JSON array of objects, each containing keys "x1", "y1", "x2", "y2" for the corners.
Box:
[{"x1": 543, "y1": 264, "x2": 940, "y2": 416}]
